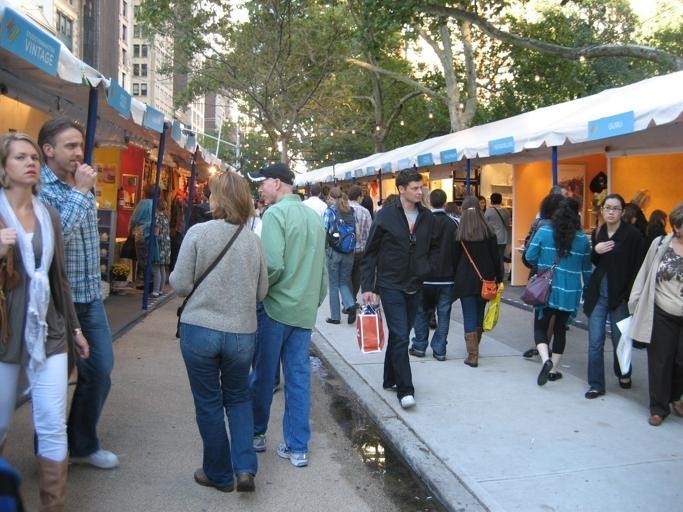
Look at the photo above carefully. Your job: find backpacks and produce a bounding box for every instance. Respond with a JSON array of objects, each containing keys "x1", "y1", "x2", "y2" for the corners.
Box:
[{"x1": 327, "y1": 207, "x2": 357, "y2": 254}]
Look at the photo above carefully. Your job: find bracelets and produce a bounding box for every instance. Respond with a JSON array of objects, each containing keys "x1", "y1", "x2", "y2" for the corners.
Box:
[{"x1": 72, "y1": 328, "x2": 82, "y2": 336}]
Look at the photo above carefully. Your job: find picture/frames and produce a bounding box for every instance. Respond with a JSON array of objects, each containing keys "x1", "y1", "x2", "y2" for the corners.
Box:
[{"x1": 121, "y1": 173, "x2": 139, "y2": 211}]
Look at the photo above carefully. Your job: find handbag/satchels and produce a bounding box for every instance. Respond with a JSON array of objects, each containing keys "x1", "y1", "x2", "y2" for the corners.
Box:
[
  {"x1": 523, "y1": 232, "x2": 537, "y2": 269},
  {"x1": 519, "y1": 269, "x2": 553, "y2": 306},
  {"x1": 131, "y1": 220, "x2": 160, "y2": 242},
  {"x1": 482, "y1": 279, "x2": 498, "y2": 300},
  {"x1": 356, "y1": 307, "x2": 385, "y2": 354},
  {"x1": 119, "y1": 232, "x2": 136, "y2": 260}
]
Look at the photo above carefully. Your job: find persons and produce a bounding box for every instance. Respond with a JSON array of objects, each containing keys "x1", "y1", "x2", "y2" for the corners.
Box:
[
  {"x1": 522, "y1": 188, "x2": 683, "y2": 426},
  {"x1": 167, "y1": 171, "x2": 270, "y2": 494},
  {"x1": 295, "y1": 180, "x2": 377, "y2": 325},
  {"x1": 126, "y1": 168, "x2": 268, "y2": 312},
  {"x1": 245, "y1": 161, "x2": 329, "y2": 468},
  {"x1": 358, "y1": 169, "x2": 430, "y2": 410},
  {"x1": 33, "y1": 118, "x2": 121, "y2": 471},
  {"x1": 410, "y1": 189, "x2": 511, "y2": 368},
  {"x1": 0, "y1": 132, "x2": 94, "y2": 512}
]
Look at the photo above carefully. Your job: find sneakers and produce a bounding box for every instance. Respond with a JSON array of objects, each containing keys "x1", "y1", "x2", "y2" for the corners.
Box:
[
  {"x1": 252, "y1": 433, "x2": 267, "y2": 452},
  {"x1": 236, "y1": 471, "x2": 256, "y2": 492},
  {"x1": 194, "y1": 468, "x2": 234, "y2": 492},
  {"x1": 400, "y1": 395, "x2": 415, "y2": 407},
  {"x1": 276, "y1": 443, "x2": 308, "y2": 467},
  {"x1": 433, "y1": 352, "x2": 445, "y2": 362},
  {"x1": 67, "y1": 448, "x2": 119, "y2": 469},
  {"x1": 325, "y1": 316, "x2": 339, "y2": 323},
  {"x1": 348, "y1": 305, "x2": 357, "y2": 325},
  {"x1": 409, "y1": 346, "x2": 424, "y2": 357}
]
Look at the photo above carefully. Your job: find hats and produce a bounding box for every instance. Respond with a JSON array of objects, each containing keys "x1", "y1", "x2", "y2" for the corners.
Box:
[{"x1": 247, "y1": 163, "x2": 296, "y2": 184}]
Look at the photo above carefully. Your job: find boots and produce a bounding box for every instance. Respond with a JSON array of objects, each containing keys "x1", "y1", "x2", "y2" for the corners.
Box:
[
  {"x1": 464, "y1": 327, "x2": 484, "y2": 368},
  {"x1": 35, "y1": 453, "x2": 70, "y2": 512}
]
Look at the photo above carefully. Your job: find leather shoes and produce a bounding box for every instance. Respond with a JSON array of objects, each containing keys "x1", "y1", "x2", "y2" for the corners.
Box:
[
  {"x1": 619, "y1": 376, "x2": 631, "y2": 388},
  {"x1": 649, "y1": 415, "x2": 664, "y2": 425},
  {"x1": 586, "y1": 390, "x2": 605, "y2": 398},
  {"x1": 672, "y1": 401, "x2": 682, "y2": 416},
  {"x1": 523, "y1": 349, "x2": 562, "y2": 385}
]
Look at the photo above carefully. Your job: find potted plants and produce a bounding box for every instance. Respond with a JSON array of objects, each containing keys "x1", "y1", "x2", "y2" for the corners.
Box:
[{"x1": 110, "y1": 263, "x2": 130, "y2": 288}]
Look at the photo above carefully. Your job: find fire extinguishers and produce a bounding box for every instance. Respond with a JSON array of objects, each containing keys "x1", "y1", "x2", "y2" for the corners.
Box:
[{"x1": 117, "y1": 186, "x2": 126, "y2": 212}]
